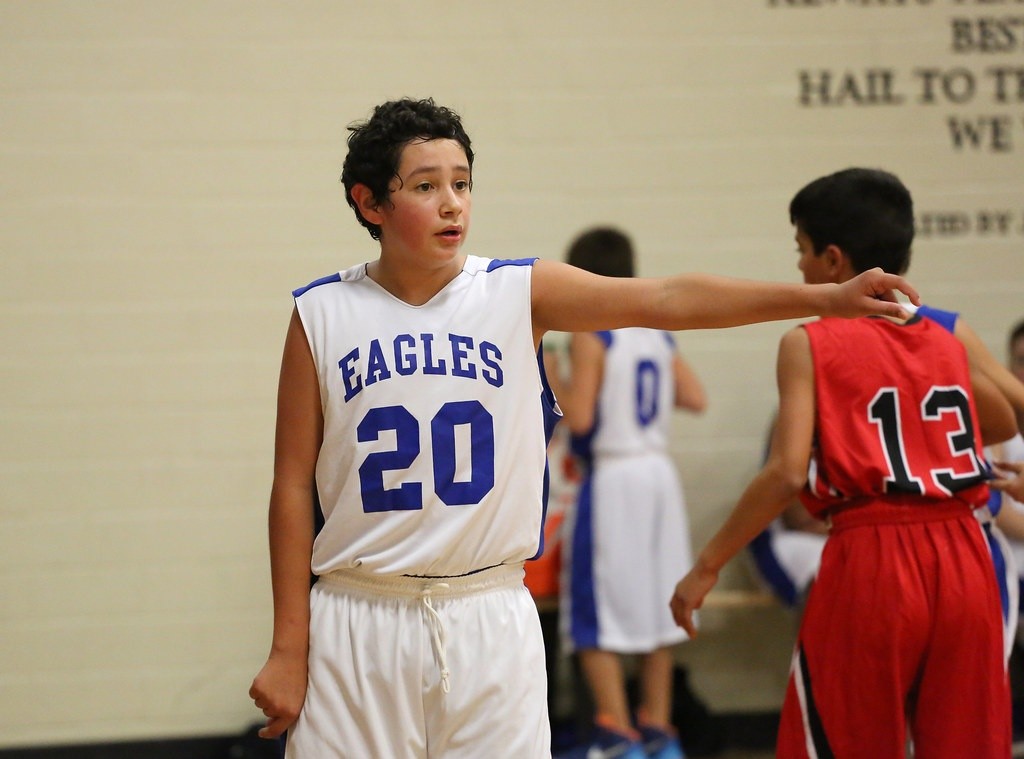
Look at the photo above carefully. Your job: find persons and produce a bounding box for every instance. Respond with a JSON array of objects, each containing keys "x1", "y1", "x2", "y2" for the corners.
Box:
[
  {"x1": 671, "y1": 167, "x2": 1018, "y2": 759},
  {"x1": 900, "y1": 302, "x2": 1024, "y2": 744},
  {"x1": 750, "y1": 416, "x2": 832, "y2": 606},
  {"x1": 249, "y1": 98, "x2": 924, "y2": 759},
  {"x1": 543, "y1": 224, "x2": 706, "y2": 759}
]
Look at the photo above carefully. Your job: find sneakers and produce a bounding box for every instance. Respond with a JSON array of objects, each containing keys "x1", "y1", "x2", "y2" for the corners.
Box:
[{"x1": 554, "y1": 718, "x2": 687, "y2": 759}]
